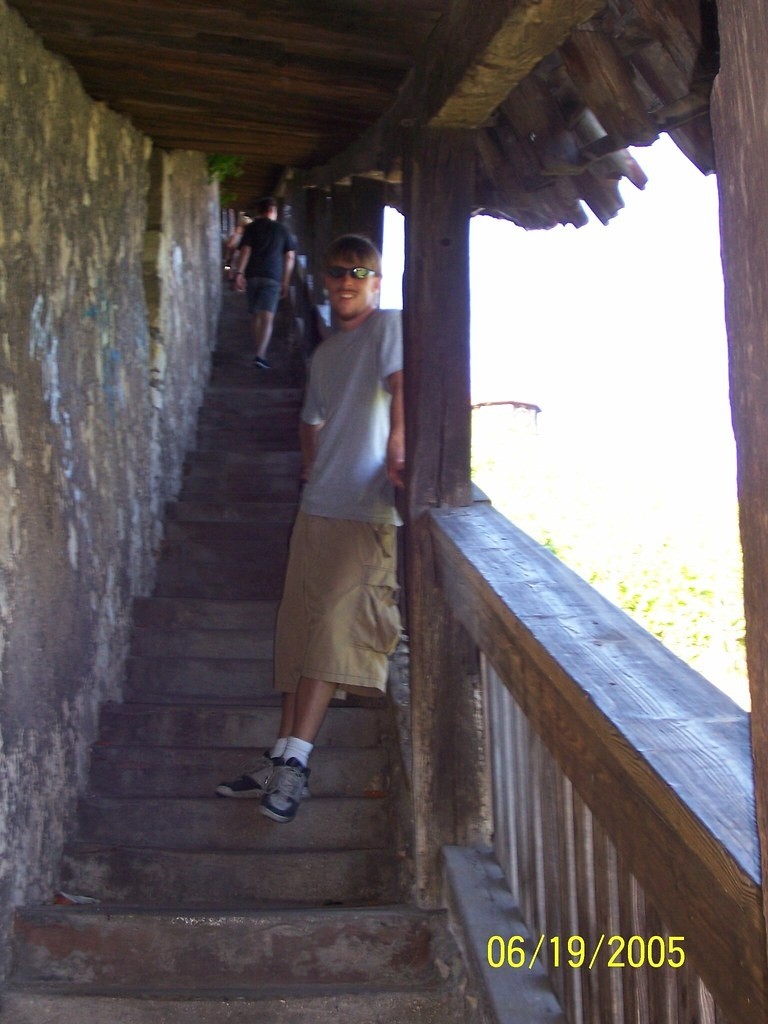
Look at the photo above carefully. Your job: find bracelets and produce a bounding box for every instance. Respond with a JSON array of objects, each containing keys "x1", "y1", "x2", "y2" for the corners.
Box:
[{"x1": 238, "y1": 271, "x2": 246, "y2": 275}]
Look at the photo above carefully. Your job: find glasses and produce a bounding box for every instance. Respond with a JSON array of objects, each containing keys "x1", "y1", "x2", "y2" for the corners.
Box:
[{"x1": 328, "y1": 266, "x2": 381, "y2": 279}]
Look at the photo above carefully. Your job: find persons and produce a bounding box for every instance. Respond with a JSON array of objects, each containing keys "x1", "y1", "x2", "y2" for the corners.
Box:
[
  {"x1": 212, "y1": 234, "x2": 408, "y2": 825},
  {"x1": 222, "y1": 196, "x2": 297, "y2": 369}
]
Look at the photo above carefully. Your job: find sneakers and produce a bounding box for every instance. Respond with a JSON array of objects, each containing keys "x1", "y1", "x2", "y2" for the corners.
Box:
[
  {"x1": 214, "y1": 751, "x2": 285, "y2": 798},
  {"x1": 258, "y1": 756, "x2": 312, "y2": 824}
]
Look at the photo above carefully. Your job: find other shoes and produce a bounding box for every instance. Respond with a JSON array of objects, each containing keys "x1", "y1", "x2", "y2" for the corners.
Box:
[{"x1": 254, "y1": 356, "x2": 271, "y2": 369}]
